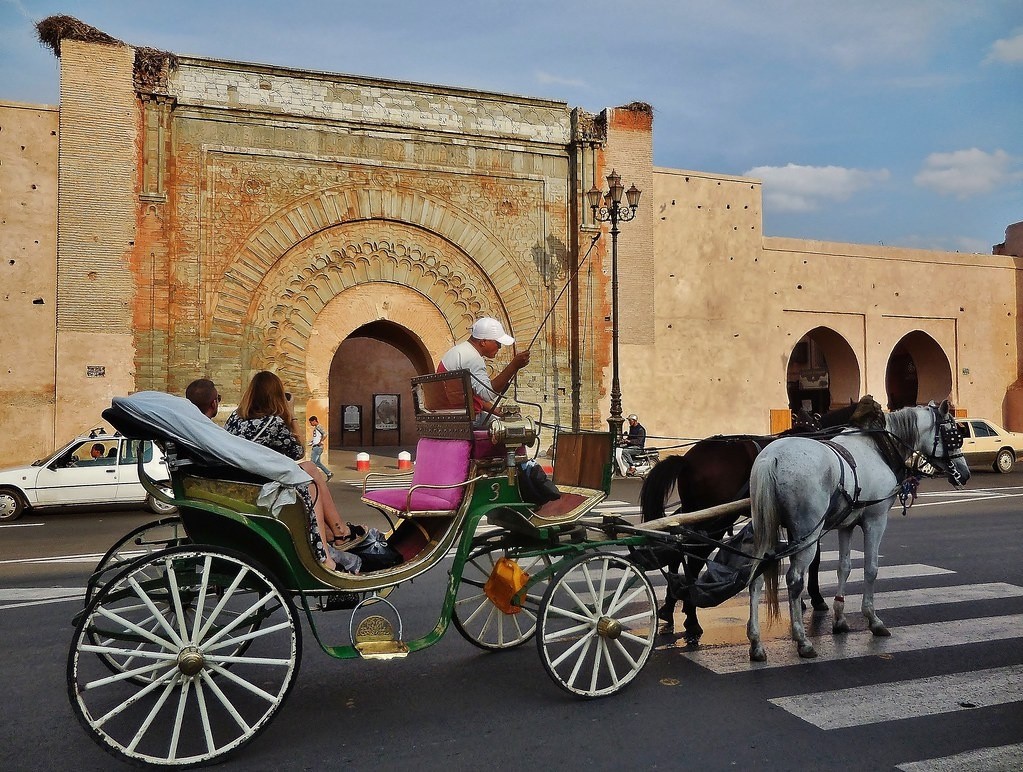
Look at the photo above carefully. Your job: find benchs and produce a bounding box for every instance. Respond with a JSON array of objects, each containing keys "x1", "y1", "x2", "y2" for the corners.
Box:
[{"x1": 359, "y1": 436, "x2": 475, "y2": 523}]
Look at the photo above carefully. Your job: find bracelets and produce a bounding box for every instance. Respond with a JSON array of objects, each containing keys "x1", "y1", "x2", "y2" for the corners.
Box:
[{"x1": 291, "y1": 418, "x2": 298, "y2": 420}]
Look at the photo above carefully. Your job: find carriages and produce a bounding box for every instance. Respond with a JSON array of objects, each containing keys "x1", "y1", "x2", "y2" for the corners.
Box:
[{"x1": 67, "y1": 367, "x2": 971, "y2": 772}]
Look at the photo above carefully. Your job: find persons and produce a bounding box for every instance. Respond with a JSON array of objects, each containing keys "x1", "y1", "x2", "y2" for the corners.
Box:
[
  {"x1": 185, "y1": 379, "x2": 221, "y2": 419},
  {"x1": 90, "y1": 444, "x2": 121, "y2": 465},
  {"x1": 438, "y1": 318, "x2": 530, "y2": 430},
  {"x1": 224, "y1": 371, "x2": 370, "y2": 551},
  {"x1": 308, "y1": 416, "x2": 335, "y2": 481},
  {"x1": 618, "y1": 413, "x2": 646, "y2": 475}
]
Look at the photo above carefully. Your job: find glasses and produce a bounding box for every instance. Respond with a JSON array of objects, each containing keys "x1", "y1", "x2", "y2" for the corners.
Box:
[
  {"x1": 209, "y1": 395, "x2": 221, "y2": 407},
  {"x1": 495, "y1": 341, "x2": 501, "y2": 349}
]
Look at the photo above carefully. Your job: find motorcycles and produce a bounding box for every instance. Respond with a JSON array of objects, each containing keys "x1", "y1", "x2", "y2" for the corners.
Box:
[{"x1": 610, "y1": 440, "x2": 659, "y2": 480}]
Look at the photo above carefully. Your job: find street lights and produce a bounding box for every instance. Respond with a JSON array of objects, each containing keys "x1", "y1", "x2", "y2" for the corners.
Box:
[{"x1": 585, "y1": 167, "x2": 642, "y2": 441}]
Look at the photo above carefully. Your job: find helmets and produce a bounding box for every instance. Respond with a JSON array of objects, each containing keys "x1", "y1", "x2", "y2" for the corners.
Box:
[{"x1": 627, "y1": 414, "x2": 638, "y2": 421}]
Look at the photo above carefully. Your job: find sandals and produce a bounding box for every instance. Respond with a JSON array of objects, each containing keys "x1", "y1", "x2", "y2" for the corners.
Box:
[
  {"x1": 327, "y1": 525, "x2": 369, "y2": 551},
  {"x1": 334, "y1": 562, "x2": 347, "y2": 573}
]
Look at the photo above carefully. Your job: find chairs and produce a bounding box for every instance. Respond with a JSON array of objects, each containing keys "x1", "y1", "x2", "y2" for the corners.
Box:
[{"x1": 409, "y1": 368, "x2": 528, "y2": 462}]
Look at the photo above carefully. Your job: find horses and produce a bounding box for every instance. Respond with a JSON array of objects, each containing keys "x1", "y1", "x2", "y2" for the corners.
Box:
[
  {"x1": 638, "y1": 397, "x2": 881, "y2": 638},
  {"x1": 747, "y1": 399, "x2": 971, "y2": 663}
]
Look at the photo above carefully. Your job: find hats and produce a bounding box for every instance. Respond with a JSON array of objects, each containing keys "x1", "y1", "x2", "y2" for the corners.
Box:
[{"x1": 469, "y1": 317, "x2": 515, "y2": 346}]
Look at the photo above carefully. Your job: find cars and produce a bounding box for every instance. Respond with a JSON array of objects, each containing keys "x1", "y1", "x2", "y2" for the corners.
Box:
[
  {"x1": 904, "y1": 417, "x2": 1023, "y2": 478},
  {"x1": 0, "y1": 427, "x2": 178, "y2": 523}
]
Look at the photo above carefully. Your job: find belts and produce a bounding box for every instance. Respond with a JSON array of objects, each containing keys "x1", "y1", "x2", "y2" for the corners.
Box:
[{"x1": 313, "y1": 444, "x2": 321, "y2": 447}]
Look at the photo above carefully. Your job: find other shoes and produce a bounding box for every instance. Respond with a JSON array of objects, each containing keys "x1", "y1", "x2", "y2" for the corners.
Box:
[
  {"x1": 627, "y1": 466, "x2": 636, "y2": 474},
  {"x1": 325, "y1": 472, "x2": 334, "y2": 482}
]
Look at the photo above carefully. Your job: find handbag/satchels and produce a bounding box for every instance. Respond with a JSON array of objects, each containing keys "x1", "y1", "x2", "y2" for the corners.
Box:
[
  {"x1": 346, "y1": 527, "x2": 403, "y2": 574},
  {"x1": 516, "y1": 458, "x2": 561, "y2": 512}
]
